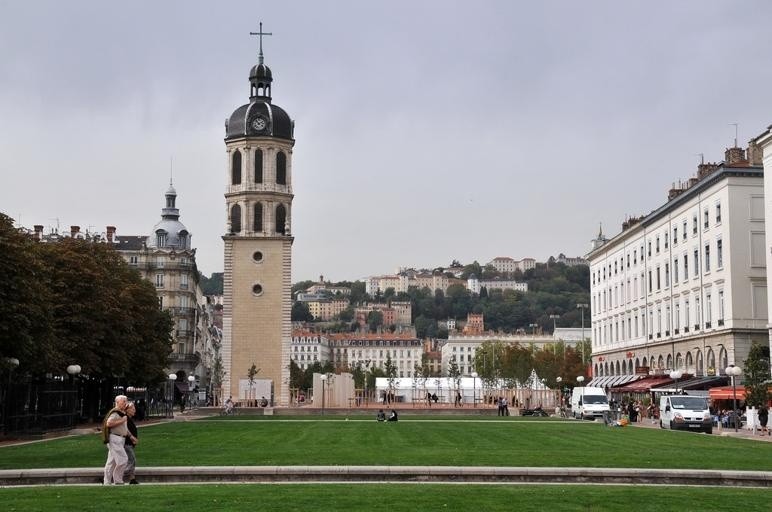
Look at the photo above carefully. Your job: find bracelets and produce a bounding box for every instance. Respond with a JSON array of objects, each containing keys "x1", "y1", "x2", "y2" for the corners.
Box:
[{"x1": 129, "y1": 433, "x2": 133, "y2": 438}]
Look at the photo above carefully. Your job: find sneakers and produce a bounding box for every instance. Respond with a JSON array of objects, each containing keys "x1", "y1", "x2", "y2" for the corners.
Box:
[{"x1": 130, "y1": 479, "x2": 138, "y2": 484}]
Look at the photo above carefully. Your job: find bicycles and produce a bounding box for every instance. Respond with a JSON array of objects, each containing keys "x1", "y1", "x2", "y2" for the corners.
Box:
[{"x1": 219, "y1": 401, "x2": 241, "y2": 416}]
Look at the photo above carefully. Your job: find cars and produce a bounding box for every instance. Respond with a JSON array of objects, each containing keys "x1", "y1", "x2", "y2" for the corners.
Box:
[
  {"x1": 712, "y1": 412, "x2": 743, "y2": 428},
  {"x1": 295, "y1": 394, "x2": 304, "y2": 402}
]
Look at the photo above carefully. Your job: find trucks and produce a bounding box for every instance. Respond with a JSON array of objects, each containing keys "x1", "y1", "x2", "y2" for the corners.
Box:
[
  {"x1": 659, "y1": 395, "x2": 712, "y2": 434},
  {"x1": 571, "y1": 386, "x2": 611, "y2": 421}
]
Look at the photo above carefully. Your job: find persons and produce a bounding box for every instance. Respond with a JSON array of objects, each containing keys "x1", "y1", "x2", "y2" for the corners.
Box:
[
  {"x1": 711, "y1": 407, "x2": 743, "y2": 429},
  {"x1": 122, "y1": 403, "x2": 139, "y2": 484},
  {"x1": 605, "y1": 393, "x2": 660, "y2": 424},
  {"x1": 102, "y1": 394, "x2": 139, "y2": 485},
  {"x1": 376, "y1": 409, "x2": 385, "y2": 422},
  {"x1": 388, "y1": 409, "x2": 398, "y2": 421},
  {"x1": 756, "y1": 401, "x2": 771, "y2": 436},
  {"x1": 259, "y1": 396, "x2": 268, "y2": 407},
  {"x1": 423, "y1": 392, "x2": 519, "y2": 416},
  {"x1": 224, "y1": 395, "x2": 233, "y2": 414}
]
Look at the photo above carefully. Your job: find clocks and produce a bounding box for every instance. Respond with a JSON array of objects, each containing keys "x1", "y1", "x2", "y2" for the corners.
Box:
[{"x1": 251, "y1": 117, "x2": 266, "y2": 132}]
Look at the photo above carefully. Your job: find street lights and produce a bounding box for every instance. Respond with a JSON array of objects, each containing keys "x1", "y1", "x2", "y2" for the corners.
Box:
[
  {"x1": 187, "y1": 371, "x2": 196, "y2": 410},
  {"x1": 576, "y1": 375, "x2": 585, "y2": 423},
  {"x1": 168, "y1": 373, "x2": 177, "y2": 416},
  {"x1": 3, "y1": 357, "x2": 22, "y2": 435},
  {"x1": 470, "y1": 372, "x2": 479, "y2": 408},
  {"x1": 555, "y1": 376, "x2": 563, "y2": 417},
  {"x1": 669, "y1": 370, "x2": 683, "y2": 394},
  {"x1": 481, "y1": 351, "x2": 488, "y2": 409},
  {"x1": 66, "y1": 363, "x2": 82, "y2": 430},
  {"x1": 320, "y1": 374, "x2": 327, "y2": 414},
  {"x1": 725, "y1": 363, "x2": 743, "y2": 433}
]
